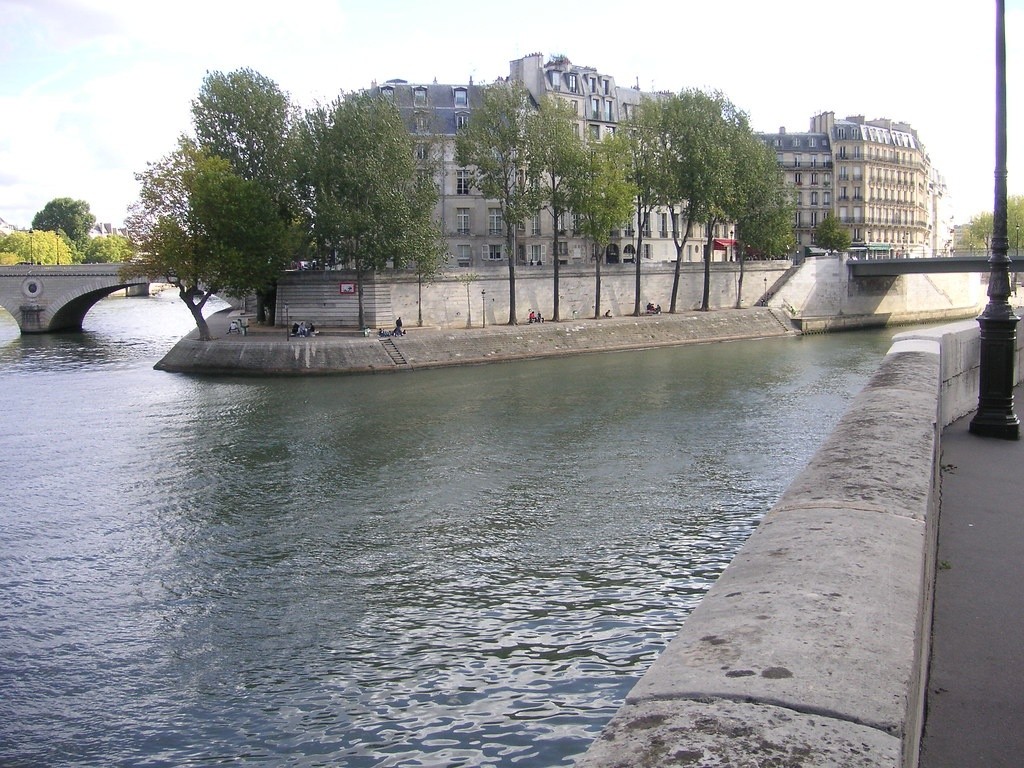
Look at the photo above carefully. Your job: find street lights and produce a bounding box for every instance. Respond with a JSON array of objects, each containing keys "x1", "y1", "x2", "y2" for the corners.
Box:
[
  {"x1": 54, "y1": 230, "x2": 60, "y2": 264},
  {"x1": 28, "y1": 228, "x2": 34, "y2": 265},
  {"x1": 1015, "y1": 223, "x2": 1020, "y2": 256}
]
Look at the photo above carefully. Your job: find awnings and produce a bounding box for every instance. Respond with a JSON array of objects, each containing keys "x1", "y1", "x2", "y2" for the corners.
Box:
[
  {"x1": 714, "y1": 238, "x2": 738, "y2": 250},
  {"x1": 809, "y1": 247, "x2": 892, "y2": 254}
]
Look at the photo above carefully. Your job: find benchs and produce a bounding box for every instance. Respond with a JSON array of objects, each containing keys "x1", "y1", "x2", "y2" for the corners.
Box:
[
  {"x1": 602, "y1": 315, "x2": 614, "y2": 319},
  {"x1": 646, "y1": 310, "x2": 662, "y2": 315},
  {"x1": 228, "y1": 320, "x2": 239, "y2": 335},
  {"x1": 528, "y1": 318, "x2": 545, "y2": 323}
]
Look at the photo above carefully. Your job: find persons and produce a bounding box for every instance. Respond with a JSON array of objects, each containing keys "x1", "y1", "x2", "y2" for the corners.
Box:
[
  {"x1": 227, "y1": 316, "x2": 403, "y2": 338},
  {"x1": 529, "y1": 258, "x2": 542, "y2": 266},
  {"x1": 529, "y1": 302, "x2": 662, "y2": 323},
  {"x1": 745, "y1": 254, "x2": 790, "y2": 261},
  {"x1": 896, "y1": 247, "x2": 955, "y2": 259}
]
